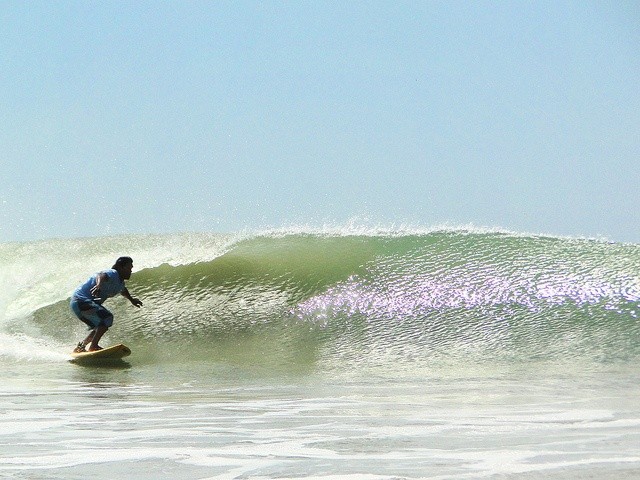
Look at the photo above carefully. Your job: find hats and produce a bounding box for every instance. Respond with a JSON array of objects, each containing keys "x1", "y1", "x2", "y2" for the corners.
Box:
[{"x1": 111, "y1": 257, "x2": 132, "y2": 269}]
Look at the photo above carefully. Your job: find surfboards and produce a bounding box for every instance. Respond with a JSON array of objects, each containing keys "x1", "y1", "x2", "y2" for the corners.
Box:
[{"x1": 74, "y1": 343, "x2": 132, "y2": 359}]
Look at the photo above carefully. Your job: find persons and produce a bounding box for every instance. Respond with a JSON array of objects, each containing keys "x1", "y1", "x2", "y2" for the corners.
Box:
[{"x1": 70, "y1": 257, "x2": 143, "y2": 353}]
